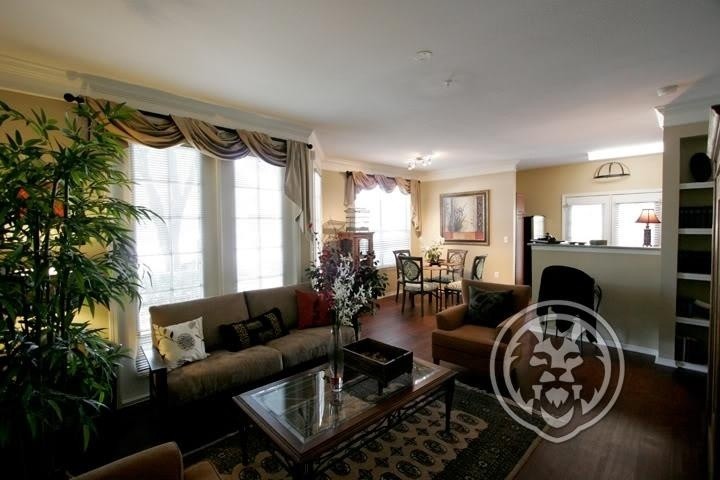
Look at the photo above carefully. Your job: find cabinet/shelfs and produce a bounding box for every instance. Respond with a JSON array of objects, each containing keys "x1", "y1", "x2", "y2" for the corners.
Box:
[
  {"x1": 336, "y1": 230, "x2": 376, "y2": 271},
  {"x1": 656, "y1": 122, "x2": 712, "y2": 371}
]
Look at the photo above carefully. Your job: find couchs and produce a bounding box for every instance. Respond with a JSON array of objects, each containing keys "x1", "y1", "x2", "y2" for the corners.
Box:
[
  {"x1": 148, "y1": 280, "x2": 358, "y2": 440},
  {"x1": 68, "y1": 442, "x2": 227, "y2": 480},
  {"x1": 432, "y1": 278, "x2": 532, "y2": 394}
]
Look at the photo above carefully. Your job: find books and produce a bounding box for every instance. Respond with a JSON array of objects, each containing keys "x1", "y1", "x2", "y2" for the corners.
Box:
[{"x1": 530, "y1": 238, "x2": 565, "y2": 244}]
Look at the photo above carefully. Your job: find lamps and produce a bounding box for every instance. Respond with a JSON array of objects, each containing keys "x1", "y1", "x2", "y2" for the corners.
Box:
[{"x1": 634, "y1": 205, "x2": 664, "y2": 247}]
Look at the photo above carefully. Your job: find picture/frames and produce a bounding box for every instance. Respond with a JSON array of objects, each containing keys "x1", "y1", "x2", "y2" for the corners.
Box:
[{"x1": 438, "y1": 190, "x2": 491, "y2": 247}]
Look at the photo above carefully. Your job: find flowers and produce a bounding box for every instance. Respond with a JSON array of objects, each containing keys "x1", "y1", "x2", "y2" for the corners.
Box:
[{"x1": 293, "y1": 207, "x2": 390, "y2": 387}]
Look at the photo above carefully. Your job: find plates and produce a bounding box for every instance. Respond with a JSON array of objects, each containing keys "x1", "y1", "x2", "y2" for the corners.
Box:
[{"x1": 530, "y1": 238, "x2": 565, "y2": 245}]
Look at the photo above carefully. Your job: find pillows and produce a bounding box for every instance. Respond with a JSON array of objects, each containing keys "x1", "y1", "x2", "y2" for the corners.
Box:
[
  {"x1": 152, "y1": 316, "x2": 212, "y2": 371},
  {"x1": 288, "y1": 287, "x2": 339, "y2": 329},
  {"x1": 466, "y1": 282, "x2": 515, "y2": 330},
  {"x1": 217, "y1": 308, "x2": 290, "y2": 349}
]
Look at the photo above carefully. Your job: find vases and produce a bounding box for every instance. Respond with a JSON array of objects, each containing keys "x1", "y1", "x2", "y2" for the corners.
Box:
[{"x1": 329, "y1": 326, "x2": 344, "y2": 393}]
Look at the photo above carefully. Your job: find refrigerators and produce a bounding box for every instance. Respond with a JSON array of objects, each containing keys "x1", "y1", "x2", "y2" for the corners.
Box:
[{"x1": 524, "y1": 214, "x2": 544, "y2": 285}]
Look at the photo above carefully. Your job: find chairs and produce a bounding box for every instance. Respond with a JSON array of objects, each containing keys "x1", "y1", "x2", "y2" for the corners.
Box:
[
  {"x1": 536, "y1": 264, "x2": 605, "y2": 359},
  {"x1": 393, "y1": 237, "x2": 487, "y2": 318}
]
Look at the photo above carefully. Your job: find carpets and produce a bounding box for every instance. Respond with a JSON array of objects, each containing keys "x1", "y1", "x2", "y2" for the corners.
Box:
[{"x1": 176, "y1": 377, "x2": 560, "y2": 479}]
